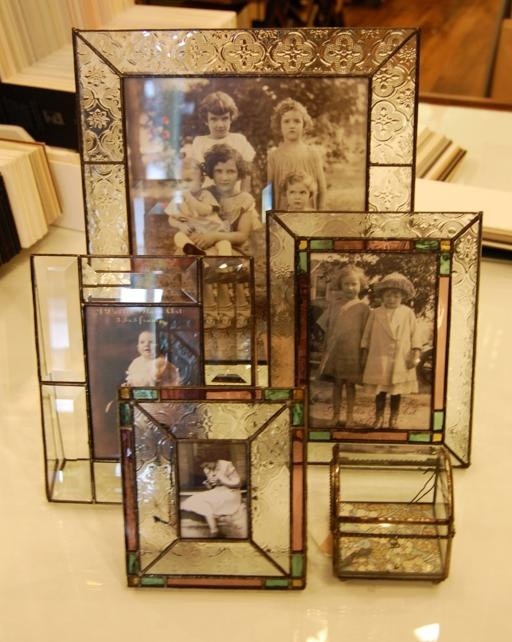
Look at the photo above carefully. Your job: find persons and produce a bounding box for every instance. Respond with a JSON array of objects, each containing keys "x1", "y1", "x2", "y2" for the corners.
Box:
[
  {"x1": 184, "y1": 91, "x2": 257, "y2": 193},
  {"x1": 179, "y1": 450, "x2": 242, "y2": 538},
  {"x1": 266, "y1": 97, "x2": 327, "y2": 210},
  {"x1": 360, "y1": 271, "x2": 422, "y2": 431},
  {"x1": 316, "y1": 264, "x2": 370, "y2": 429},
  {"x1": 174, "y1": 142, "x2": 256, "y2": 256},
  {"x1": 163, "y1": 158, "x2": 232, "y2": 256},
  {"x1": 126, "y1": 330, "x2": 180, "y2": 388},
  {"x1": 278, "y1": 170, "x2": 318, "y2": 211}
]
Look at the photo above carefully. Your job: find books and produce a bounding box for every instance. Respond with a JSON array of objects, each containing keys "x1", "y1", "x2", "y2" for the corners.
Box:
[{"x1": 0, "y1": 138, "x2": 63, "y2": 266}]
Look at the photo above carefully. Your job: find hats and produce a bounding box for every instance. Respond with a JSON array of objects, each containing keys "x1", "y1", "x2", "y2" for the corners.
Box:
[{"x1": 374, "y1": 272, "x2": 416, "y2": 302}]
